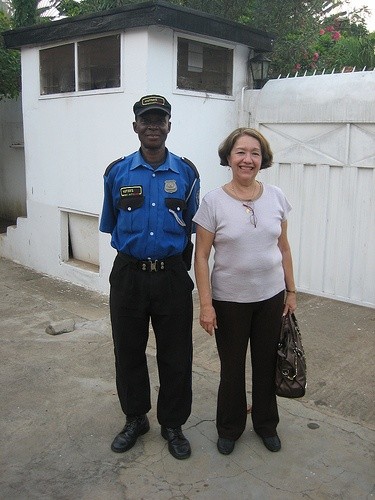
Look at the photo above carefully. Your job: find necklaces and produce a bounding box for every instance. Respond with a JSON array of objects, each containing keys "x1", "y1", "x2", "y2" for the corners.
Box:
[{"x1": 231, "y1": 182, "x2": 256, "y2": 214}]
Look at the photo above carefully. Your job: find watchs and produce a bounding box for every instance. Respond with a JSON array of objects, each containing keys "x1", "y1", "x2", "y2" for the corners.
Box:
[{"x1": 286, "y1": 290, "x2": 297, "y2": 294}]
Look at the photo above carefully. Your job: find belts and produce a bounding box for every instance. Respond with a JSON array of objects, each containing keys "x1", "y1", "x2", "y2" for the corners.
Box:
[{"x1": 137, "y1": 259, "x2": 172, "y2": 273}]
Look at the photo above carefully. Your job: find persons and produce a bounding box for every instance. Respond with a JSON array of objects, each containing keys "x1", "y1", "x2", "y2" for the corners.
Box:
[
  {"x1": 192, "y1": 126, "x2": 307, "y2": 455},
  {"x1": 97, "y1": 94, "x2": 201, "y2": 460}
]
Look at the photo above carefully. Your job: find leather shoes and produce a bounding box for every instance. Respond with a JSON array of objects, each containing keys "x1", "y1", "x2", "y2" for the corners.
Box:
[
  {"x1": 161, "y1": 427, "x2": 191, "y2": 460},
  {"x1": 111, "y1": 415, "x2": 150, "y2": 453},
  {"x1": 253, "y1": 424, "x2": 281, "y2": 452},
  {"x1": 217, "y1": 436, "x2": 234, "y2": 454}
]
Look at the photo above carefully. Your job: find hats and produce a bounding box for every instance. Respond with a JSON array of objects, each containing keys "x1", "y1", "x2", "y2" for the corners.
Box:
[{"x1": 134, "y1": 95, "x2": 171, "y2": 120}]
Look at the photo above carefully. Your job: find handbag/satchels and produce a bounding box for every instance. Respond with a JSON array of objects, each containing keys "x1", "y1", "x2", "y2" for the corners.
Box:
[{"x1": 274, "y1": 309, "x2": 306, "y2": 397}]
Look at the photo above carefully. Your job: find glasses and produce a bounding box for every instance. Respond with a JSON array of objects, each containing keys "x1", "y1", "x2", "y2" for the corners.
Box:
[{"x1": 243, "y1": 200, "x2": 257, "y2": 228}]
